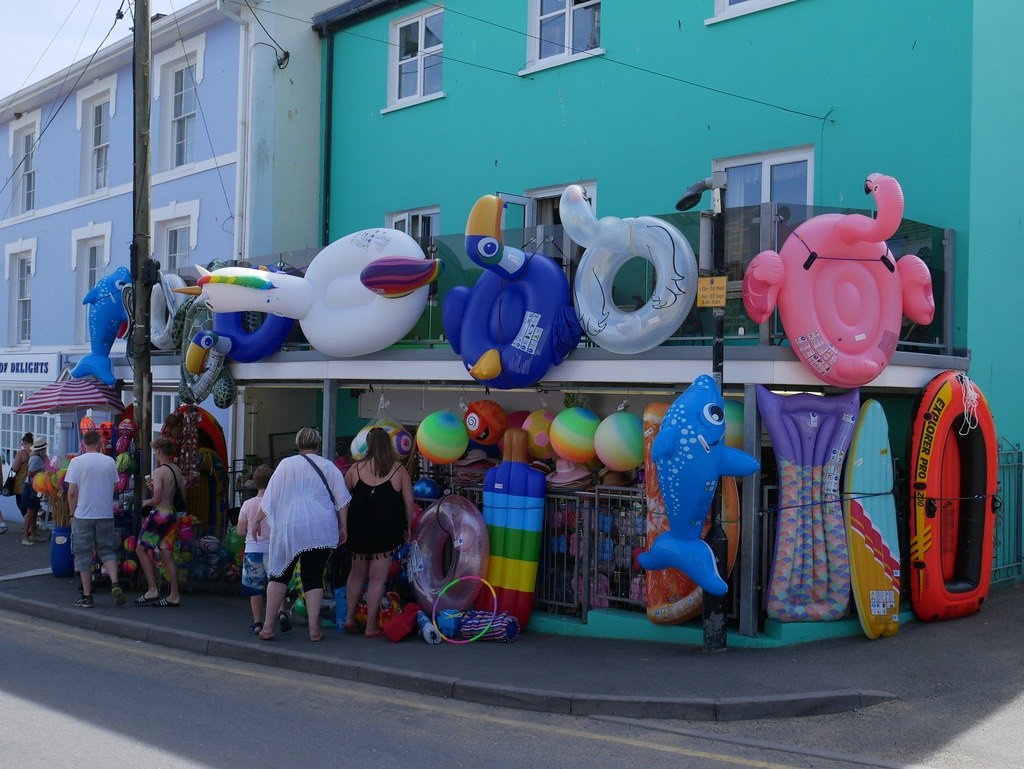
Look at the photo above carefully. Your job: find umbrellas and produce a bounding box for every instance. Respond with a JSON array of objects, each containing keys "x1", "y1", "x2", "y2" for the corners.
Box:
[{"x1": 14, "y1": 375, "x2": 128, "y2": 454}]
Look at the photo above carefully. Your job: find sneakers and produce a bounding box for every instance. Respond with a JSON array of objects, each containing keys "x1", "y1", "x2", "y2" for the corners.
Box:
[
  {"x1": 74, "y1": 594, "x2": 95, "y2": 608},
  {"x1": 112, "y1": 582, "x2": 127, "y2": 607}
]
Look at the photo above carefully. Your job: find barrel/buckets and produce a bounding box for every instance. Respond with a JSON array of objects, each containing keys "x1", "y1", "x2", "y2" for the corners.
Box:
[{"x1": 50, "y1": 527, "x2": 73, "y2": 578}]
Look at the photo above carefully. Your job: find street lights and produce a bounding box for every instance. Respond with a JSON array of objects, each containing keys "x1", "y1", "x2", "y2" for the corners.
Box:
[{"x1": 677, "y1": 171, "x2": 730, "y2": 652}]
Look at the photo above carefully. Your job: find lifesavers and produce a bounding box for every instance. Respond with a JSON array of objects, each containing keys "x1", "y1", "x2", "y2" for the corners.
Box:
[
  {"x1": 408, "y1": 492, "x2": 495, "y2": 609},
  {"x1": 131, "y1": 275, "x2": 298, "y2": 407},
  {"x1": 557, "y1": 185, "x2": 700, "y2": 351}
]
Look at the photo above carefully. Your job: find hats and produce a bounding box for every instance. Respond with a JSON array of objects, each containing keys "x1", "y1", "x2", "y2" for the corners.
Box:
[{"x1": 30, "y1": 438, "x2": 49, "y2": 450}]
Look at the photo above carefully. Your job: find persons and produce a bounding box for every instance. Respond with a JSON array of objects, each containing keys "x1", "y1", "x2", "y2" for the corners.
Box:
[
  {"x1": 133, "y1": 436, "x2": 183, "y2": 607},
  {"x1": 0, "y1": 448, "x2": 8, "y2": 534},
  {"x1": 237, "y1": 465, "x2": 293, "y2": 634},
  {"x1": 21, "y1": 437, "x2": 46, "y2": 546},
  {"x1": 11, "y1": 432, "x2": 36, "y2": 535},
  {"x1": 64, "y1": 431, "x2": 127, "y2": 607},
  {"x1": 345, "y1": 428, "x2": 413, "y2": 638},
  {"x1": 252, "y1": 428, "x2": 352, "y2": 641}
]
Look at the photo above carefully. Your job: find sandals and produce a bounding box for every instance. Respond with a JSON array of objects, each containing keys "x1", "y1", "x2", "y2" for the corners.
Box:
[
  {"x1": 278, "y1": 611, "x2": 291, "y2": 631},
  {"x1": 249, "y1": 621, "x2": 263, "y2": 635}
]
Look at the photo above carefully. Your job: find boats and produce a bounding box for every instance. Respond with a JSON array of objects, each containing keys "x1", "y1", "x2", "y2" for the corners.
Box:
[
  {"x1": 901, "y1": 370, "x2": 1003, "y2": 623},
  {"x1": 157, "y1": 404, "x2": 229, "y2": 541}
]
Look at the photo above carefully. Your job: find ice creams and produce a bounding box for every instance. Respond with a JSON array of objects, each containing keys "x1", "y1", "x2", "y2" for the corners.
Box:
[{"x1": 145, "y1": 474, "x2": 151, "y2": 483}]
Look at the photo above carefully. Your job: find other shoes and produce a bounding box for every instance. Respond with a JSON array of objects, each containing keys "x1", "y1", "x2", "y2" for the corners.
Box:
[
  {"x1": 363, "y1": 630, "x2": 384, "y2": 638},
  {"x1": 0, "y1": 526, "x2": 7, "y2": 533},
  {"x1": 29, "y1": 535, "x2": 47, "y2": 542},
  {"x1": 344, "y1": 624, "x2": 361, "y2": 634},
  {"x1": 22, "y1": 540, "x2": 34, "y2": 545}
]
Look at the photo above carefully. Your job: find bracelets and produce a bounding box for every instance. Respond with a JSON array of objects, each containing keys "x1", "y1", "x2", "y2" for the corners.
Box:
[{"x1": 69, "y1": 514, "x2": 74, "y2": 517}]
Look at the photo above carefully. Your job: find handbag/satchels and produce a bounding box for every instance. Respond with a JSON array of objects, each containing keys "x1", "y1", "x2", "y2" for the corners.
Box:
[
  {"x1": 172, "y1": 487, "x2": 186, "y2": 513},
  {"x1": 1, "y1": 468, "x2": 16, "y2": 497}
]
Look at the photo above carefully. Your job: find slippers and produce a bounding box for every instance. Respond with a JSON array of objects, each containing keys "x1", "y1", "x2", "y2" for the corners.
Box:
[
  {"x1": 153, "y1": 598, "x2": 180, "y2": 606},
  {"x1": 310, "y1": 632, "x2": 322, "y2": 641},
  {"x1": 134, "y1": 595, "x2": 159, "y2": 605},
  {"x1": 259, "y1": 630, "x2": 274, "y2": 639}
]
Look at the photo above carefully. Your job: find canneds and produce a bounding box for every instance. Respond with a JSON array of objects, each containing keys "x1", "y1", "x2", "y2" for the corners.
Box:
[{"x1": 124, "y1": 502, "x2": 128, "y2": 510}]
